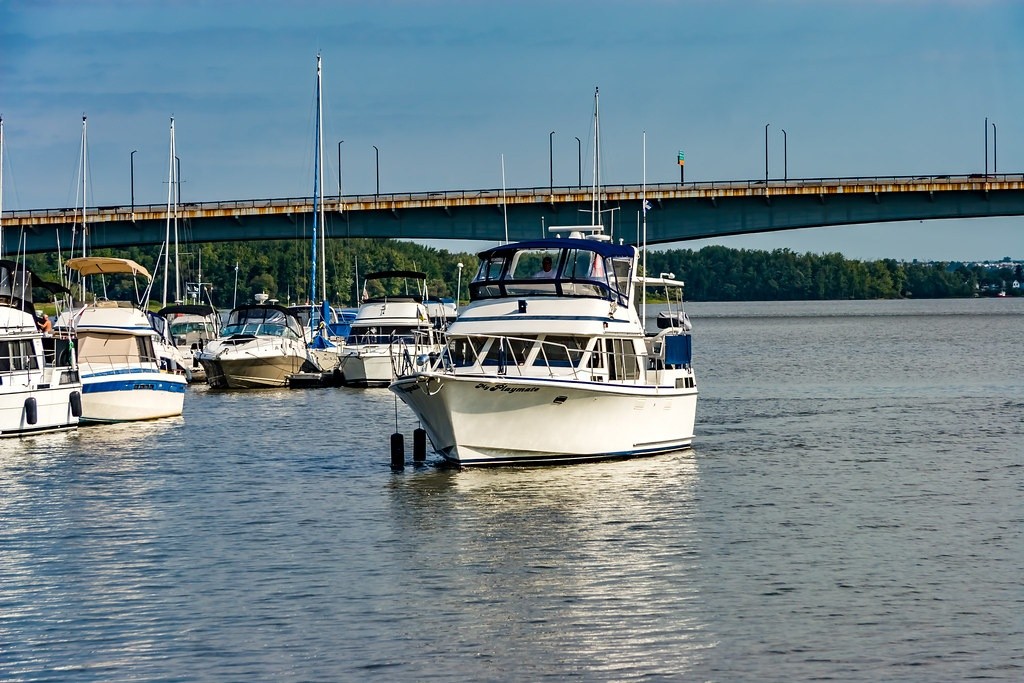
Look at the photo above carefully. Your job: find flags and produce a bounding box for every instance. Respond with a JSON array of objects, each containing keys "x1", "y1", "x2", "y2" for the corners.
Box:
[
  {"x1": 363, "y1": 290, "x2": 368, "y2": 300},
  {"x1": 416, "y1": 308, "x2": 423, "y2": 321},
  {"x1": 645, "y1": 200, "x2": 653, "y2": 210}
]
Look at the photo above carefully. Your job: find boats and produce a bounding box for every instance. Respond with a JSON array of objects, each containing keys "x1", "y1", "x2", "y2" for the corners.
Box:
[
  {"x1": 386, "y1": 80, "x2": 700, "y2": 469},
  {"x1": 0, "y1": 48, "x2": 450, "y2": 439}
]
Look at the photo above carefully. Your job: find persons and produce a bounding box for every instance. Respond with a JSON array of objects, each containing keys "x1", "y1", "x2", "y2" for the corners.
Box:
[
  {"x1": 37, "y1": 315, "x2": 52, "y2": 334},
  {"x1": 533, "y1": 257, "x2": 562, "y2": 278}
]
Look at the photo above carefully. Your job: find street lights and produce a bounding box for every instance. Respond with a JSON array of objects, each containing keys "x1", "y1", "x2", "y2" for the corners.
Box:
[
  {"x1": 338, "y1": 140, "x2": 345, "y2": 200},
  {"x1": 984, "y1": 117, "x2": 989, "y2": 178},
  {"x1": 765, "y1": 123, "x2": 770, "y2": 185},
  {"x1": 991, "y1": 123, "x2": 996, "y2": 178},
  {"x1": 575, "y1": 136, "x2": 581, "y2": 189},
  {"x1": 549, "y1": 131, "x2": 555, "y2": 195},
  {"x1": 372, "y1": 145, "x2": 379, "y2": 198},
  {"x1": 782, "y1": 128, "x2": 787, "y2": 182},
  {"x1": 130, "y1": 150, "x2": 137, "y2": 207}
]
